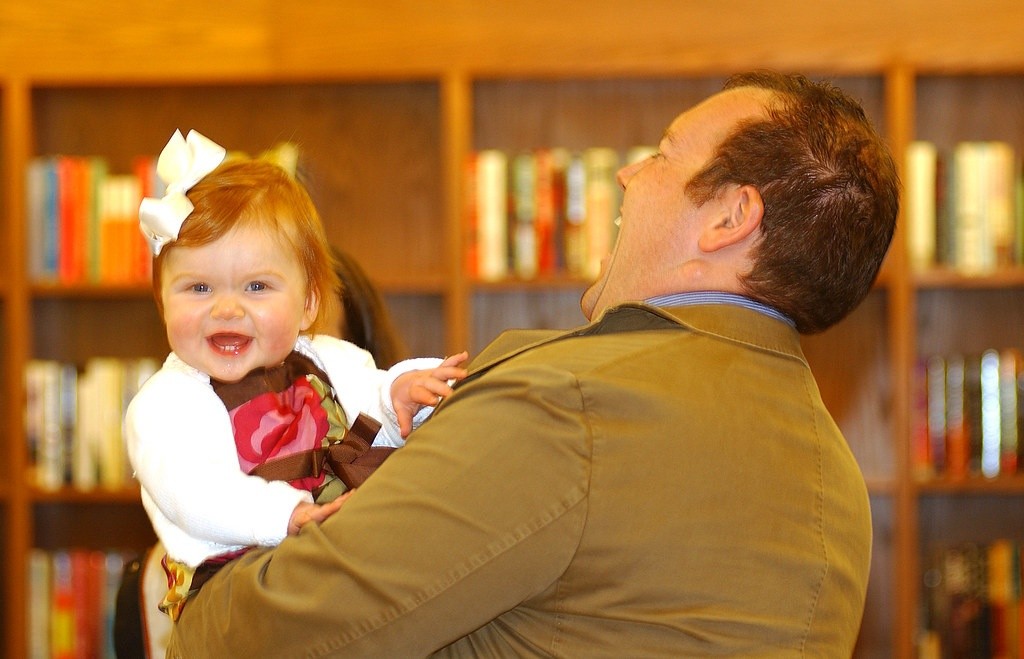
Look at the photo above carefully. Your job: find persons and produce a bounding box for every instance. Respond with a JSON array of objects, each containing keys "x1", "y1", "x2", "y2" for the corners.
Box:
[
  {"x1": 156, "y1": 70, "x2": 903, "y2": 659},
  {"x1": 299, "y1": 243, "x2": 410, "y2": 373},
  {"x1": 124, "y1": 126, "x2": 467, "y2": 568}
]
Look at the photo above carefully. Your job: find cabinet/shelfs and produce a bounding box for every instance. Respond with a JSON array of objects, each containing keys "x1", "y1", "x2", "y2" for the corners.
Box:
[{"x1": 2, "y1": 1, "x2": 1024, "y2": 658}]
